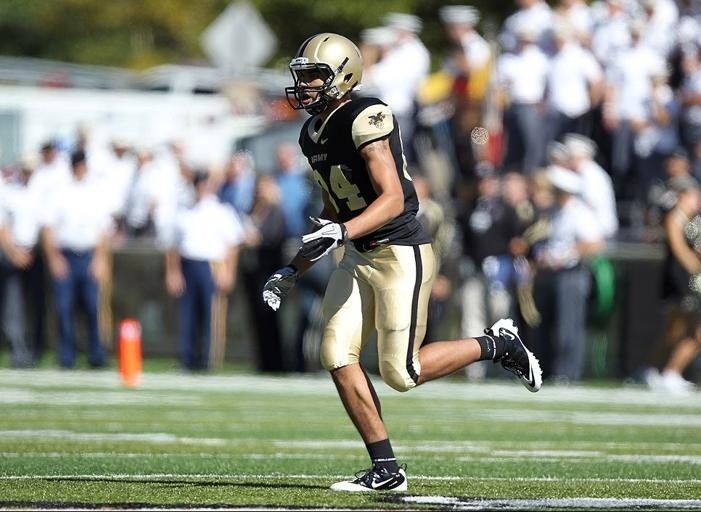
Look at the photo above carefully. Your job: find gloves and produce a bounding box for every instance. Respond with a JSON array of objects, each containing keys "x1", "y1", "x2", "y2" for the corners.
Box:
[
  {"x1": 262, "y1": 264, "x2": 301, "y2": 312},
  {"x1": 299, "y1": 216, "x2": 348, "y2": 262}
]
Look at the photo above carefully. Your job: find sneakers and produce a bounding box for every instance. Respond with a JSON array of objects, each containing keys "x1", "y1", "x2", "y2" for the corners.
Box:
[
  {"x1": 330, "y1": 463, "x2": 408, "y2": 493},
  {"x1": 484, "y1": 316, "x2": 543, "y2": 392}
]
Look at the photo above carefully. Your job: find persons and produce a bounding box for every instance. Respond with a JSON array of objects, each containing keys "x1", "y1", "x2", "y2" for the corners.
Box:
[
  {"x1": 0, "y1": 130, "x2": 336, "y2": 373},
  {"x1": 263, "y1": 32, "x2": 543, "y2": 491},
  {"x1": 355, "y1": 0, "x2": 701, "y2": 389}
]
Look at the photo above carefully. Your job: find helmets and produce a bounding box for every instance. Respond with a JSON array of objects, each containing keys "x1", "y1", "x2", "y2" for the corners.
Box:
[{"x1": 288, "y1": 32, "x2": 363, "y2": 100}]
